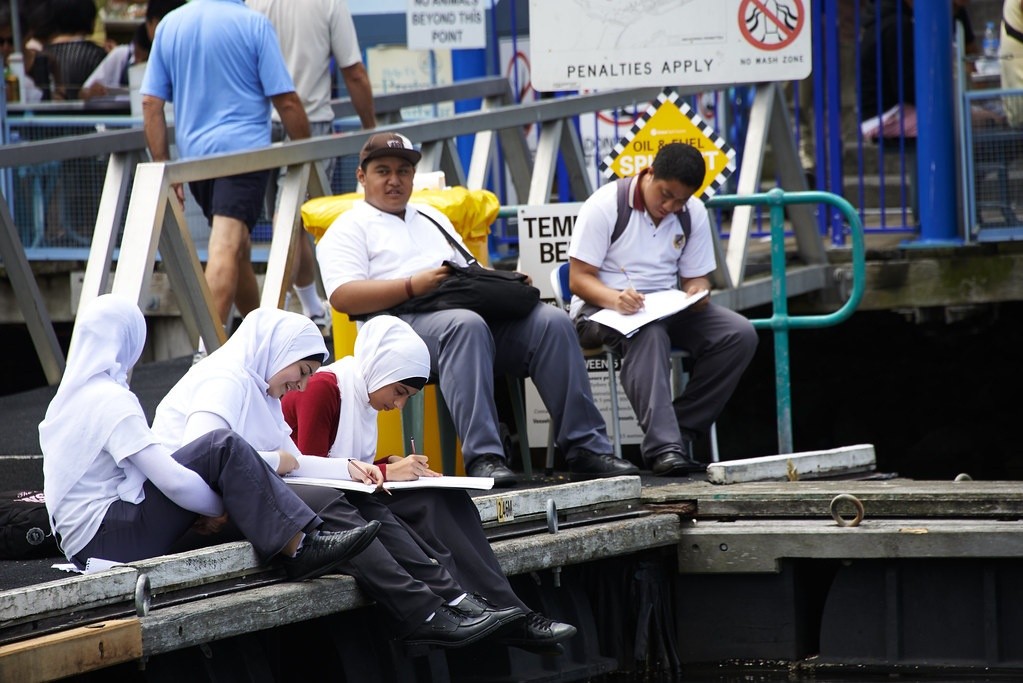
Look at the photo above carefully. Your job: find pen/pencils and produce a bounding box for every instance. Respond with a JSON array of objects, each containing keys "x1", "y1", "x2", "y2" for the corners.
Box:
[
  {"x1": 620, "y1": 266, "x2": 645, "y2": 310},
  {"x1": 348, "y1": 459, "x2": 393, "y2": 496},
  {"x1": 410, "y1": 437, "x2": 416, "y2": 454}
]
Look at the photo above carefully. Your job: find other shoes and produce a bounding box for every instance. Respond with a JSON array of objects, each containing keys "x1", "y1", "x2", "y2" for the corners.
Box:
[{"x1": 312, "y1": 302, "x2": 331, "y2": 335}]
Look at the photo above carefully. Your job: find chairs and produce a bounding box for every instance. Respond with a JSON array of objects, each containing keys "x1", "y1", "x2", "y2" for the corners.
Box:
[
  {"x1": 309, "y1": 234, "x2": 533, "y2": 483},
  {"x1": 544, "y1": 261, "x2": 720, "y2": 477}
]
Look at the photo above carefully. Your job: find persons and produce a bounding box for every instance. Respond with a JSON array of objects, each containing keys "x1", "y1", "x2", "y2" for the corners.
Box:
[
  {"x1": 316, "y1": 133, "x2": 643, "y2": 483},
  {"x1": 569, "y1": 142, "x2": 758, "y2": 473},
  {"x1": 142, "y1": 0, "x2": 312, "y2": 368},
  {"x1": 150, "y1": 307, "x2": 526, "y2": 646},
  {"x1": 280, "y1": 314, "x2": 577, "y2": 646},
  {"x1": 243, "y1": 0, "x2": 378, "y2": 206},
  {"x1": 37, "y1": 294, "x2": 380, "y2": 581},
  {"x1": 82, "y1": 5, "x2": 172, "y2": 97}
]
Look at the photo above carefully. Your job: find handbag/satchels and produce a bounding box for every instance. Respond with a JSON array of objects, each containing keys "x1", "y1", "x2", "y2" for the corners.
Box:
[{"x1": 351, "y1": 263, "x2": 540, "y2": 322}]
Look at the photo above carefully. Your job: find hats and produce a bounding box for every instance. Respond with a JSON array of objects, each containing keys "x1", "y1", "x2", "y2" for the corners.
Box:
[
  {"x1": 302, "y1": 352, "x2": 324, "y2": 366},
  {"x1": 361, "y1": 132, "x2": 420, "y2": 168},
  {"x1": 401, "y1": 376, "x2": 427, "y2": 390}
]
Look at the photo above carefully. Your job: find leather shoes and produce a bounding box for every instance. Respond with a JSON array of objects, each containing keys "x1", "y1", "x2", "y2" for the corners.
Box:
[
  {"x1": 566, "y1": 448, "x2": 639, "y2": 480},
  {"x1": 317, "y1": 520, "x2": 381, "y2": 556},
  {"x1": 467, "y1": 455, "x2": 517, "y2": 487},
  {"x1": 511, "y1": 632, "x2": 563, "y2": 656},
  {"x1": 490, "y1": 614, "x2": 576, "y2": 646},
  {"x1": 650, "y1": 451, "x2": 689, "y2": 476},
  {"x1": 280, "y1": 527, "x2": 366, "y2": 581},
  {"x1": 444, "y1": 593, "x2": 526, "y2": 637},
  {"x1": 402, "y1": 606, "x2": 505, "y2": 656},
  {"x1": 684, "y1": 451, "x2": 709, "y2": 473}
]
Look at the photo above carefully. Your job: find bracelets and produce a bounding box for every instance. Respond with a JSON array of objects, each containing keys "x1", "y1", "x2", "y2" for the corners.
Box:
[{"x1": 405, "y1": 276, "x2": 414, "y2": 299}]
